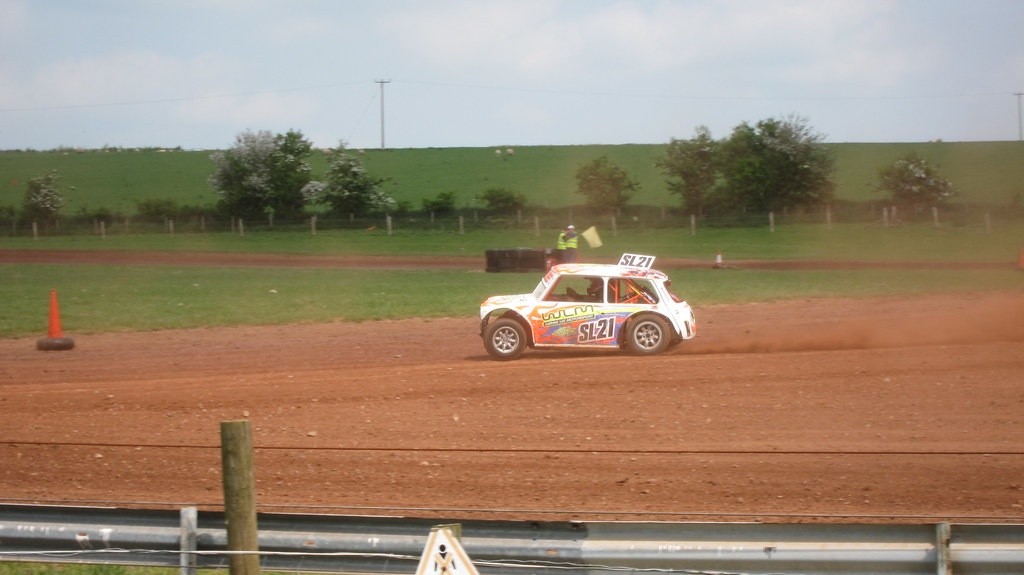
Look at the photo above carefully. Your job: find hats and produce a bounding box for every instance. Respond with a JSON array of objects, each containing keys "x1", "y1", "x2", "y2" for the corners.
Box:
[{"x1": 567, "y1": 225, "x2": 575, "y2": 228}]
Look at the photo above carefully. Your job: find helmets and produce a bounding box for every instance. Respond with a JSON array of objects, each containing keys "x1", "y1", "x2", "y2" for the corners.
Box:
[{"x1": 584, "y1": 276, "x2": 604, "y2": 295}]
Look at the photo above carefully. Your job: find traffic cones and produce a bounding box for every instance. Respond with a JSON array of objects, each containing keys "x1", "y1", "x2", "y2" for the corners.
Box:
[{"x1": 48, "y1": 288, "x2": 62, "y2": 338}]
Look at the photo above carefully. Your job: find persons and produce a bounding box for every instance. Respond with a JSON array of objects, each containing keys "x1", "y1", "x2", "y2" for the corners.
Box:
[
  {"x1": 566, "y1": 277, "x2": 603, "y2": 302},
  {"x1": 565, "y1": 225, "x2": 578, "y2": 263},
  {"x1": 557, "y1": 228, "x2": 569, "y2": 263}
]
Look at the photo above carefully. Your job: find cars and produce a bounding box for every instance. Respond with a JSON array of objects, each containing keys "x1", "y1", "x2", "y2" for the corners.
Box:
[{"x1": 477, "y1": 261, "x2": 699, "y2": 359}]
